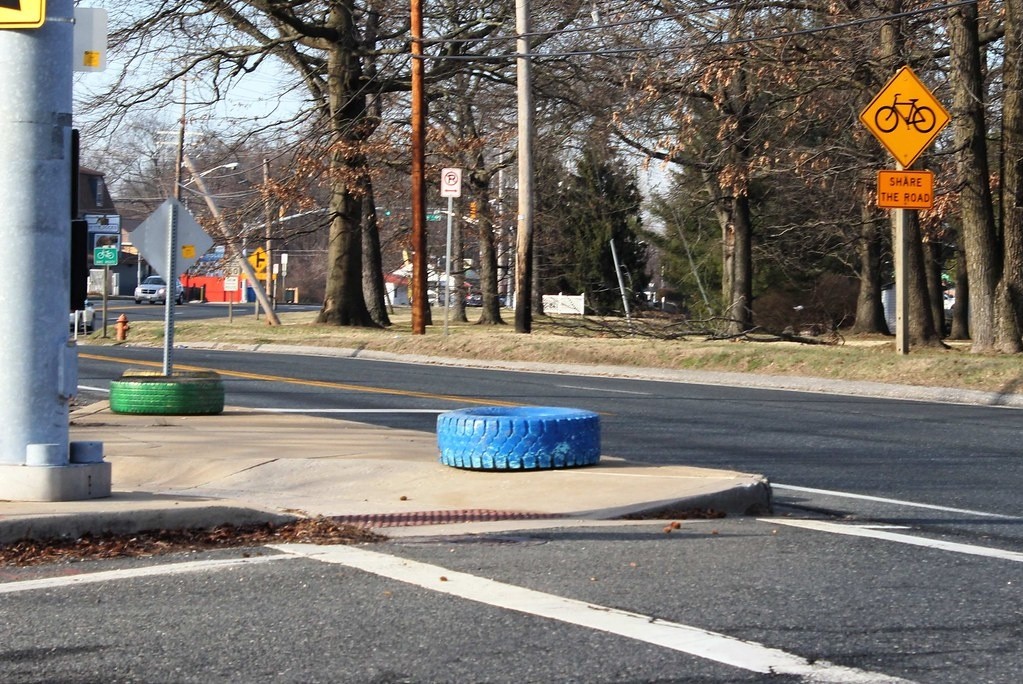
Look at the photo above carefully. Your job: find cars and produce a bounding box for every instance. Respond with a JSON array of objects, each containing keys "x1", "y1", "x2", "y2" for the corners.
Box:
[
  {"x1": 880, "y1": 271, "x2": 960, "y2": 338},
  {"x1": 133, "y1": 275, "x2": 186, "y2": 306},
  {"x1": 405, "y1": 276, "x2": 483, "y2": 308},
  {"x1": 69, "y1": 298, "x2": 96, "y2": 333},
  {"x1": 631, "y1": 280, "x2": 664, "y2": 306}
]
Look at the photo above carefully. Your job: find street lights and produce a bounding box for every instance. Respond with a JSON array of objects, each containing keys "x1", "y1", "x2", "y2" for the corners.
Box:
[{"x1": 168, "y1": 161, "x2": 241, "y2": 324}]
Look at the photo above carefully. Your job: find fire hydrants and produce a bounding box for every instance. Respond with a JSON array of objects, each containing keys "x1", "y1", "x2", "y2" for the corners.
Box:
[{"x1": 115, "y1": 312, "x2": 130, "y2": 341}]
